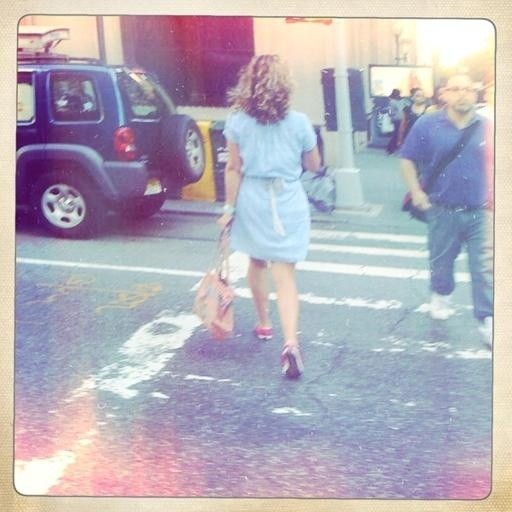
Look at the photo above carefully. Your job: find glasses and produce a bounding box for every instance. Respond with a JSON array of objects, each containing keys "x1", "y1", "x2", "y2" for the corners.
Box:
[{"x1": 443, "y1": 86, "x2": 475, "y2": 93}]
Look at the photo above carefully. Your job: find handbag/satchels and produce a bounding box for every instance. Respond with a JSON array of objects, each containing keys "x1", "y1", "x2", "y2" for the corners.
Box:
[
  {"x1": 401, "y1": 180, "x2": 430, "y2": 223},
  {"x1": 383, "y1": 135, "x2": 397, "y2": 156},
  {"x1": 375, "y1": 114, "x2": 394, "y2": 135},
  {"x1": 300, "y1": 169, "x2": 338, "y2": 214},
  {"x1": 191, "y1": 226, "x2": 234, "y2": 343}
]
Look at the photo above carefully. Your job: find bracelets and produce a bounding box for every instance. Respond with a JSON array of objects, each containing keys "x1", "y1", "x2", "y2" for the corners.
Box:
[{"x1": 222, "y1": 205, "x2": 236, "y2": 214}]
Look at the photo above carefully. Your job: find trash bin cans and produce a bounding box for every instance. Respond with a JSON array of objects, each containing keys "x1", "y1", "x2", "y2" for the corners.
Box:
[{"x1": 178, "y1": 121, "x2": 229, "y2": 201}]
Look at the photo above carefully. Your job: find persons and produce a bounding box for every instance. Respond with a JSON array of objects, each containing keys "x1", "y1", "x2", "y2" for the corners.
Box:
[
  {"x1": 193, "y1": 55, "x2": 321, "y2": 380},
  {"x1": 386, "y1": 75, "x2": 494, "y2": 349}
]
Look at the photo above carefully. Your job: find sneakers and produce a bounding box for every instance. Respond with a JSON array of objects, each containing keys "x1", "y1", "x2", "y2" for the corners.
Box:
[
  {"x1": 281, "y1": 343, "x2": 304, "y2": 377},
  {"x1": 430, "y1": 288, "x2": 456, "y2": 319},
  {"x1": 252, "y1": 323, "x2": 273, "y2": 340},
  {"x1": 479, "y1": 316, "x2": 493, "y2": 350}
]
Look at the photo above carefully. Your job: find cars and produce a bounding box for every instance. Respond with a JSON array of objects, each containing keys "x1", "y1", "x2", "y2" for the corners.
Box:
[{"x1": 16, "y1": 56, "x2": 206, "y2": 238}]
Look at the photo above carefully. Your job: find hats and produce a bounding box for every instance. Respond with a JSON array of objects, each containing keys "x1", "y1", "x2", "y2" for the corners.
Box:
[{"x1": 389, "y1": 88, "x2": 403, "y2": 100}]
